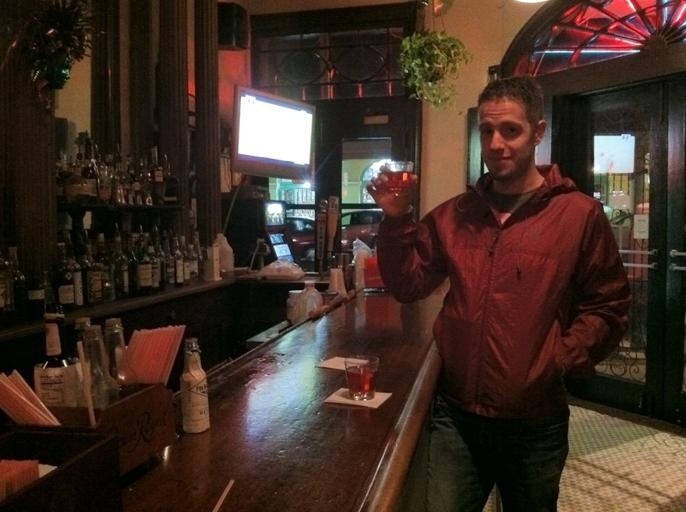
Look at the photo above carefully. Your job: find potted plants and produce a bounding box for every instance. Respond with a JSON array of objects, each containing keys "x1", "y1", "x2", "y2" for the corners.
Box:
[{"x1": 396, "y1": 28, "x2": 472, "y2": 109}]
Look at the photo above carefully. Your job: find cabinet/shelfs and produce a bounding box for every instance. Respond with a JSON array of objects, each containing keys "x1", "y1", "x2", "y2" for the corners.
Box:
[
  {"x1": 237, "y1": 284, "x2": 334, "y2": 357},
  {"x1": 0, "y1": 278, "x2": 235, "y2": 401}
]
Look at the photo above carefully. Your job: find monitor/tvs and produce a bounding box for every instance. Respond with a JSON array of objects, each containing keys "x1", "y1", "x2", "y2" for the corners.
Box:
[
  {"x1": 230, "y1": 85, "x2": 319, "y2": 178},
  {"x1": 259, "y1": 200, "x2": 289, "y2": 227}
]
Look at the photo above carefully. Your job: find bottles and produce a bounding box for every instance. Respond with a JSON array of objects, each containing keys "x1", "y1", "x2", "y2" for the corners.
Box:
[
  {"x1": 287, "y1": 280, "x2": 323, "y2": 325},
  {"x1": 57, "y1": 212, "x2": 200, "y2": 309},
  {"x1": 0, "y1": 250, "x2": 46, "y2": 330},
  {"x1": 32, "y1": 310, "x2": 134, "y2": 408},
  {"x1": 178, "y1": 337, "x2": 211, "y2": 435},
  {"x1": 56, "y1": 140, "x2": 179, "y2": 209},
  {"x1": 329, "y1": 251, "x2": 357, "y2": 296}
]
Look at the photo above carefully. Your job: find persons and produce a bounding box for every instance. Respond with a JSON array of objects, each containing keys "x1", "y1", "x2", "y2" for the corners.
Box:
[{"x1": 366, "y1": 74, "x2": 633, "y2": 512}]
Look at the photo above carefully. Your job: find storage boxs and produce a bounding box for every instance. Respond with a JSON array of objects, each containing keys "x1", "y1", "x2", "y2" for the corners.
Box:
[
  {"x1": 48, "y1": 380, "x2": 173, "y2": 494},
  {"x1": 0, "y1": 422, "x2": 124, "y2": 511}
]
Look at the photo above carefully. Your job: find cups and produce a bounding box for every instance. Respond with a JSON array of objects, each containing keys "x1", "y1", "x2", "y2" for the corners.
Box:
[
  {"x1": 384, "y1": 161, "x2": 414, "y2": 195},
  {"x1": 344, "y1": 355, "x2": 380, "y2": 401}
]
